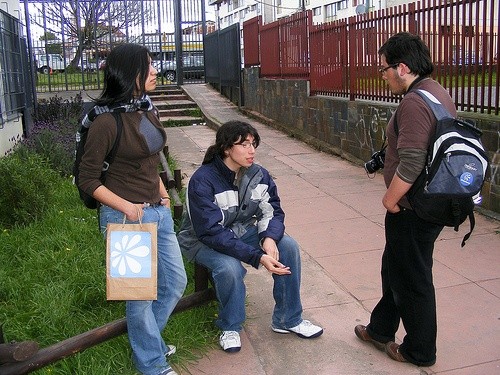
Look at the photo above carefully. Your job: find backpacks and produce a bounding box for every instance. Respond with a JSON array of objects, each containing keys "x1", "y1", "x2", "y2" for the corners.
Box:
[
  {"x1": 393, "y1": 88, "x2": 490, "y2": 248},
  {"x1": 72, "y1": 111, "x2": 123, "y2": 210}
]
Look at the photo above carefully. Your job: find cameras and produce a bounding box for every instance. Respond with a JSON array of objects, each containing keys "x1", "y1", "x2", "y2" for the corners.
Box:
[{"x1": 364, "y1": 150, "x2": 385, "y2": 174}]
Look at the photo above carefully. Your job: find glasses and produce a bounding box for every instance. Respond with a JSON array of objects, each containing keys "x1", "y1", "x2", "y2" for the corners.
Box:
[
  {"x1": 379, "y1": 63, "x2": 400, "y2": 75},
  {"x1": 233, "y1": 141, "x2": 258, "y2": 149}
]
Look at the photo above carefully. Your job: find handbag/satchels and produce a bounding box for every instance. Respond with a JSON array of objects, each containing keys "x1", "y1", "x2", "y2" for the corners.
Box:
[{"x1": 104, "y1": 204, "x2": 158, "y2": 301}]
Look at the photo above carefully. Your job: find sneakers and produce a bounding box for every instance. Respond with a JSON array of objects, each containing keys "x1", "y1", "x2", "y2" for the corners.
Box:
[
  {"x1": 271, "y1": 319, "x2": 324, "y2": 339},
  {"x1": 384, "y1": 340, "x2": 409, "y2": 362},
  {"x1": 219, "y1": 330, "x2": 242, "y2": 352},
  {"x1": 354, "y1": 324, "x2": 386, "y2": 351}
]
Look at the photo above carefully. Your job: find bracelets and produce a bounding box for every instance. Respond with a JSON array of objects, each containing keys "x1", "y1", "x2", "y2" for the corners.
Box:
[{"x1": 162, "y1": 197, "x2": 171, "y2": 200}]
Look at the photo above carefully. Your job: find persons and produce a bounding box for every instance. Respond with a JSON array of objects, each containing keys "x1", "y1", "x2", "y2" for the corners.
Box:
[
  {"x1": 77, "y1": 42, "x2": 189, "y2": 375},
  {"x1": 354, "y1": 31, "x2": 457, "y2": 367},
  {"x1": 176, "y1": 121, "x2": 323, "y2": 352}
]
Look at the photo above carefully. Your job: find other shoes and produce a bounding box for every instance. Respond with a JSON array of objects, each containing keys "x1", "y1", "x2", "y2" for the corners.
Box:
[
  {"x1": 163, "y1": 345, "x2": 177, "y2": 357},
  {"x1": 158, "y1": 366, "x2": 178, "y2": 375}
]
